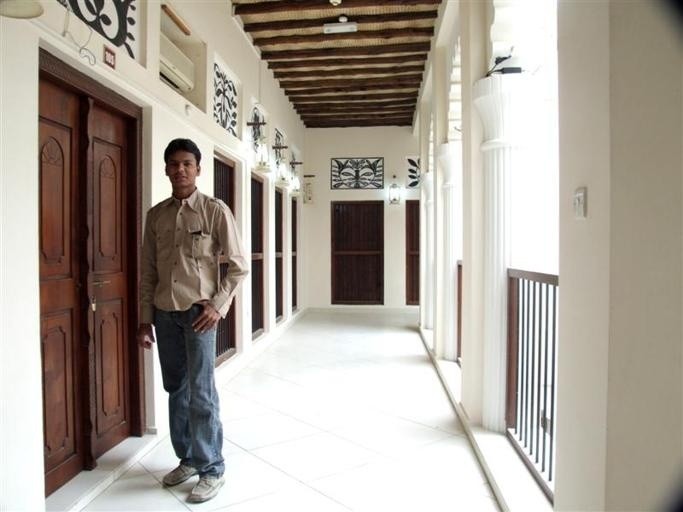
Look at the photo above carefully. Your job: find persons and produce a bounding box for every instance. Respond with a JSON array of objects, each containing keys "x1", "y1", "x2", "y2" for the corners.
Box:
[{"x1": 134, "y1": 137, "x2": 246, "y2": 503}]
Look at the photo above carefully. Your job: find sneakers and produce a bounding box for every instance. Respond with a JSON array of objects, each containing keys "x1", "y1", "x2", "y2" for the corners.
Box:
[
  {"x1": 163, "y1": 463, "x2": 198, "y2": 486},
  {"x1": 190, "y1": 474, "x2": 226, "y2": 503}
]
